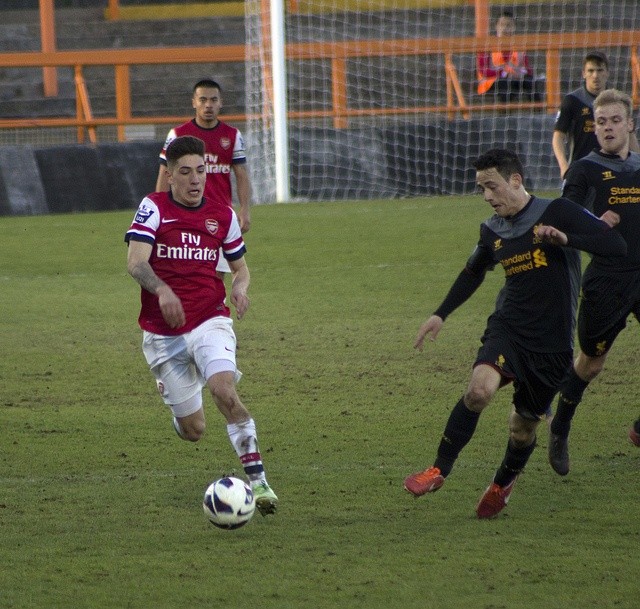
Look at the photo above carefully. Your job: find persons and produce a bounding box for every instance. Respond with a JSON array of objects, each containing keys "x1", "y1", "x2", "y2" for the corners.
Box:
[
  {"x1": 155, "y1": 80, "x2": 253, "y2": 294},
  {"x1": 403, "y1": 149, "x2": 628, "y2": 521},
  {"x1": 550, "y1": 88, "x2": 639, "y2": 477},
  {"x1": 473, "y1": 12, "x2": 541, "y2": 115},
  {"x1": 128, "y1": 135, "x2": 282, "y2": 517},
  {"x1": 552, "y1": 51, "x2": 632, "y2": 187}
]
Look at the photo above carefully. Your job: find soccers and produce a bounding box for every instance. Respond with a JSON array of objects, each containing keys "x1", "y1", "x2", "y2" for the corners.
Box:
[{"x1": 202, "y1": 478, "x2": 255, "y2": 530}]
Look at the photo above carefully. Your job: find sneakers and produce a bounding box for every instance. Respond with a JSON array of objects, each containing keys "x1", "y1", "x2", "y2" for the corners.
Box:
[
  {"x1": 628, "y1": 429, "x2": 640, "y2": 447},
  {"x1": 475, "y1": 473, "x2": 519, "y2": 518},
  {"x1": 548, "y1": 430, "x2": 569, "y2": 475},
  {"x1": 252, "y1": 479, "x2": 280, "y2": 518},
  {"x1": 403, "y1": 465, "x2": 447, "y2": 497}
]
